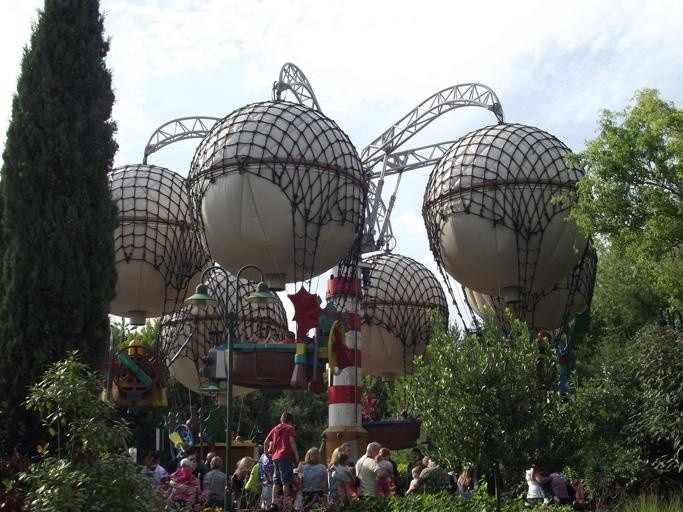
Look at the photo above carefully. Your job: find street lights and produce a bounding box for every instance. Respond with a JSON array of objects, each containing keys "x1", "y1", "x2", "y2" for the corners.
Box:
[{"x1": 183, "y1": 264, "x2": 278, "y2": 512}]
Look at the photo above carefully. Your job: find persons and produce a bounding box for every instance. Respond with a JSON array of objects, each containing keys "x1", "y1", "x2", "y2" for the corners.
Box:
[
  {"x1": 525, "y1": 461, "x2": 590, "y2": 512},
  {"x1": 230, "y1": 430, "x2": 274, "y2": 512},
  {"x1": 264, "y1": 413, "x2": 299, "y2": 512},
  {"x1": 293, "y1": 442, "x2": 400, "y2": 510},
  {"x1": 144, "y1": 418, "x2": 225, "y2": 512},
  {"x1": 404, "y1": 448, "x2": 474, "y2": 498}
]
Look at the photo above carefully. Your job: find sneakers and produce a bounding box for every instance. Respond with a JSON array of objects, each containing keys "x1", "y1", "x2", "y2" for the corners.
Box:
[{"x1": 270, "y1": 503, "x2": 278, "y2": 511}]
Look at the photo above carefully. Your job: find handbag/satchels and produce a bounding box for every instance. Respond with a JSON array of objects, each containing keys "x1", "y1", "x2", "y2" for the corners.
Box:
[
  {"x1": 292, "y1": 490, "x2": 304, "y2": 511},
  {"x1": 244, "y1": 463, "x2": 261, "y2": 494},
  {"x1": 353, "y1": 477, "x2": 359, "y2": 487}
]
